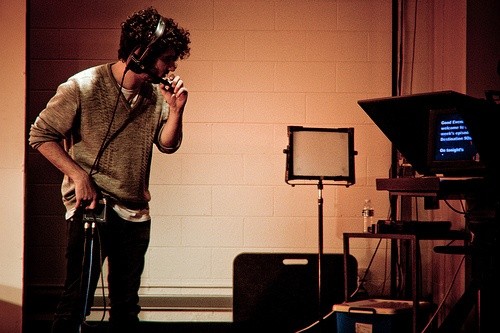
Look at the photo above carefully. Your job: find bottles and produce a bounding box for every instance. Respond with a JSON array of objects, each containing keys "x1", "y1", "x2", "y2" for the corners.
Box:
[{"x1": 362, "y1": 199, "x2": 374, "y2": 233}]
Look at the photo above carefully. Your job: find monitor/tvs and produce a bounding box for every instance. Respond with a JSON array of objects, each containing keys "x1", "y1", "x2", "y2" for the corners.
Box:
[{"x1": 429, "y1": 109, "x2": 483, "y2": 172}]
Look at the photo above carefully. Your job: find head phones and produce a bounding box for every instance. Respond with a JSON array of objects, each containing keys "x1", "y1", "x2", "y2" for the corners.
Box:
[{"x1": 126, "y1": 14, "x2": 165, "y2": 74}]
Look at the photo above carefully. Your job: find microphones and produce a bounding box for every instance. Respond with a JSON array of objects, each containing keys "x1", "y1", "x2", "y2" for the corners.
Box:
[{"x1": 142, "y1": 70, "x2": 173, "y2": 87}]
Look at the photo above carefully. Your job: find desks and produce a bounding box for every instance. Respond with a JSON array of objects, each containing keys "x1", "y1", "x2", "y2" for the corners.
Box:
[{"x1": 342, "y1": 230, "x2": 476, "y2": 333}]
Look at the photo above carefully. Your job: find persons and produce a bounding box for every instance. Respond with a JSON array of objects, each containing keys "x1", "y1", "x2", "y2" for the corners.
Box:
[{"x1": 30, "y1": 5, "x2": 191, "y2": 333}]
